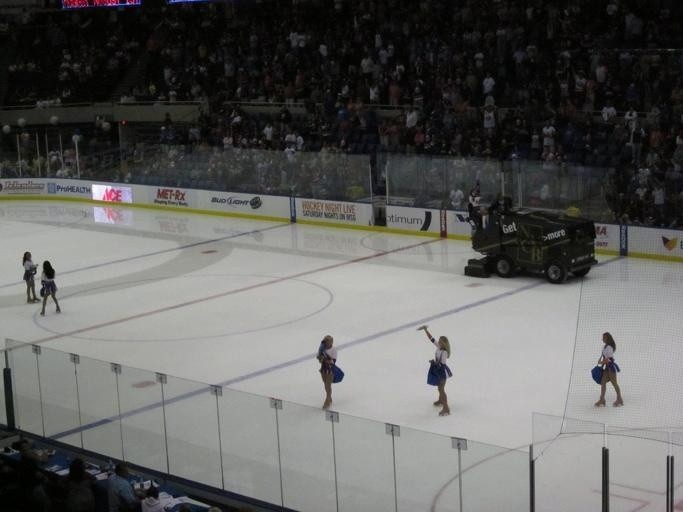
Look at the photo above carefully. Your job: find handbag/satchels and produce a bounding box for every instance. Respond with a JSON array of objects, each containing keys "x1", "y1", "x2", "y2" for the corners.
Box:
[
  {"x1": 591, "y1": 366, "x2": 602, "y2": 384},
  {"x1": 332, "y1": 365, "x2": 344, "y2": 383},
  {"x1": 40, "y1": 288, "x2": 45, "y2": 296},
  {"x1": 427, "y1": 365, "x2": 440, "y2": 386}
]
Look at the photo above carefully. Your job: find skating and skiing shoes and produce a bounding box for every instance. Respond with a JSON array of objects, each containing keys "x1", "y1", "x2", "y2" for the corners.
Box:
[
  {"x1": 56, "y1": 308, "x2": 60, "y2": 312},
  {"x1": 439, "y1": 409, "x2": 450, "y2": 416},
  {"x1": 594, "y1": 399, "x2": 607, "y2": 407},
  {"x1": 27, "y1": 298, "x2": 41, "y2": 304},
  {"x1": 323, "y1": 398, "x2": 332, "y2": 408},
  {"x1": 613, "y1": 399, "x2": 624, "y2": 407},
  {"x1": 434, "y1": 401, "x2": 443, "y2": 406},
  {"x1": 40, "y1": 312, "x2": 45, "y2": 315}
]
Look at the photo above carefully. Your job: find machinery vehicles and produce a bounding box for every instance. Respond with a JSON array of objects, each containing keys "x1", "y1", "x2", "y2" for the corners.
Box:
[{"x1": 465, "y1": 195, "x2": 599, "y2": 284}]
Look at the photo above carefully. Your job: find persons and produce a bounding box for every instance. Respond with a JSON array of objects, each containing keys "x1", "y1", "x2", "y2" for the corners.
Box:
[
  {"x1": 39, "y1": 260, "x2": 61, "y2": 316},
  {"x1": 21, "y1": 251, "x2": 42, "y2": 304},
  {"x1": 0, "y1": 433, "x2": 225, "y2": 512},
  {"x1": 592, "y1": 331, "x2": 623, "y2": 407},
  {"x1": 418, "y1": 324, "x2": 450, "y2": 415},
  {"x1": 316, "y1": 335, "x2": 337, "y2": 412}
]
